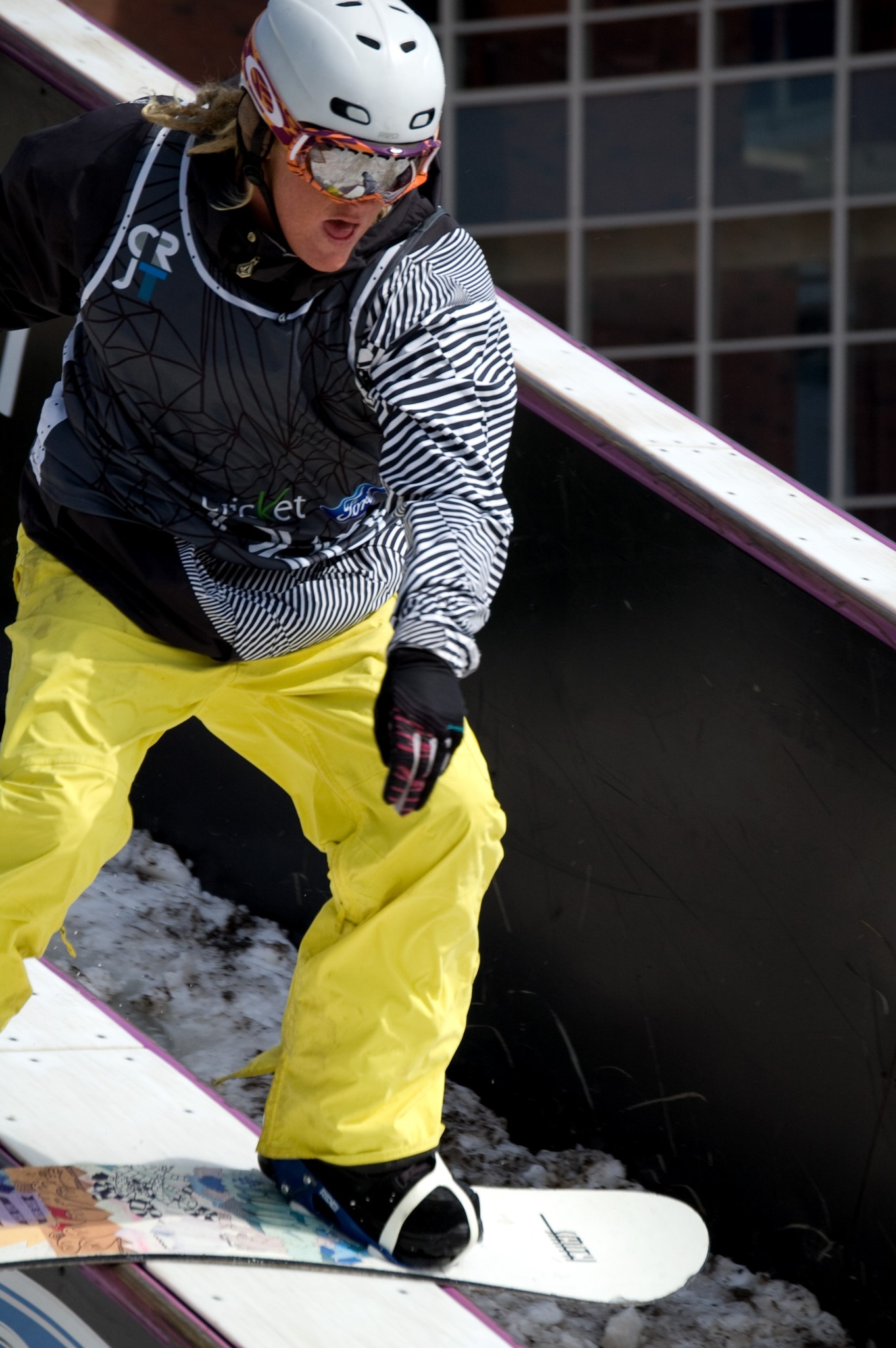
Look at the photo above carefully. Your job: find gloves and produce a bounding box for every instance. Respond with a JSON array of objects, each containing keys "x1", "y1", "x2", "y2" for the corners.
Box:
[{"x1": 374, "y1": 643, "x2": 469, "y2": 822}]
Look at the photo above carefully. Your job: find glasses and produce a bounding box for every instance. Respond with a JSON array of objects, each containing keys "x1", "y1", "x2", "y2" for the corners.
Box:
[{"x1": 285, "y1": 128, "x2": 444, "y2": 206}]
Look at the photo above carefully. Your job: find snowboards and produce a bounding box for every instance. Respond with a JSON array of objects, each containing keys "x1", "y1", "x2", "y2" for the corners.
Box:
[{"x1": 1, "y1": 1160, "x2": 711, "y2": 1304}]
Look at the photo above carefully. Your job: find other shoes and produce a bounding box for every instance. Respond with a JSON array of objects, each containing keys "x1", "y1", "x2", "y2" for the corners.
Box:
[{"x1": 253, "y1": 1134, "x2": 481, "y2": 1260}]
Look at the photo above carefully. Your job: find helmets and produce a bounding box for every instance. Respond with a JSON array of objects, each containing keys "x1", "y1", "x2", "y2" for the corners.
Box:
[{"x1": 251, "y1": 0, "x2": 445, "y2": 151}]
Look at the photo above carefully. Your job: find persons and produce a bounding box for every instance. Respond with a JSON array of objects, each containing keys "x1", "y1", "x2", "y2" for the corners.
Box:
[{"x1": 0, "y1": 0, "x2": 525, "y2": 1276}]
